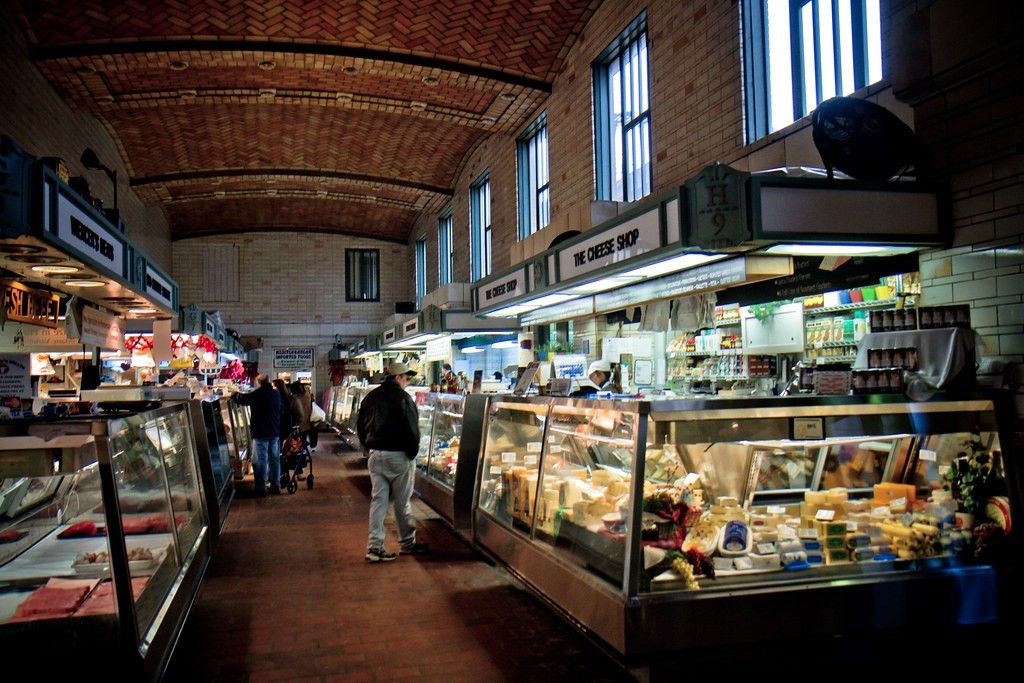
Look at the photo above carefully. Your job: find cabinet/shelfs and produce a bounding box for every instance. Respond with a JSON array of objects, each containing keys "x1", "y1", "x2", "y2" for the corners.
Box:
[
  {"x1": 1, "y1": 378, "x2": 252, "y2": 683},
  {"x1": 322, "y1": 283, "x2": 1023, "y2": 683}
]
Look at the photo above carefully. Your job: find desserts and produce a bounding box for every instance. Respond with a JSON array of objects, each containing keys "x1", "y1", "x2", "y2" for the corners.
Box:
[{"x1": 484, "y1": 461, "x2": 977, "y2": 573}]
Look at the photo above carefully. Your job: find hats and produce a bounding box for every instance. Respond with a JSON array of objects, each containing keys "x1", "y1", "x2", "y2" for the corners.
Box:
[
  {"x1": 384, "y1": 362, "x2": 417, "y2": 376},
  {"x1": 586, "y1": 360, "x2": 610, "y2": 376}
]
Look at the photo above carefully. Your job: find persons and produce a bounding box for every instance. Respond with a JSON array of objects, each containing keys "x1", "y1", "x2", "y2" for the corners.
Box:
[
  {"x1": 434, "y1": 385, "x2": 440, "y2": 393},
  {"x1": 226, "y1": 374, "x2": 283, "y2": 496},
  {"x1": 429, "y1": 384, "x2": 434, "y2": 392},
  {"x1": 290, "y1": 381, "x2": 313, "y2": 444},
  {"x1": 441, "y1": 363, "x2": 459, "y2": 394},
  {"x1": 588, "y1": 361, "x2": 623, "y2": 396},
  {"x1": 270, "y1": 380, "x2": 304, "y2": 442},
  {"x1": 357, "y1": 363, "x2": 420, "y2": 562}
]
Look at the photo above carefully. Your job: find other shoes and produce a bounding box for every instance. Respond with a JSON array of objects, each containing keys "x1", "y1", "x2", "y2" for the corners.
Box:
[
  {"x1": 254, "y1": 489, "x2": 266, "y2": 497},
  {"x1": 267, "y1": 486, "x2": 282, "y2": 494}
]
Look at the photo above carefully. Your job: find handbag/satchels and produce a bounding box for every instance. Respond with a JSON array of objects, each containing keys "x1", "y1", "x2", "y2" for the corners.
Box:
[{"x1": 310, "y1": 401, "x2": 326, "y2": 427}]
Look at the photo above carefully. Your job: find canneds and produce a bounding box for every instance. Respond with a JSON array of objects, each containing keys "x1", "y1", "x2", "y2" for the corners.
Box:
[{"x1": 853, "y1": 305, "x2": 968, "y2": 392}]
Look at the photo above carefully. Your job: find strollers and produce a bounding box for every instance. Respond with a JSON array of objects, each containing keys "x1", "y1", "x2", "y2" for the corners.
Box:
[{"x1": 279, "y1": 419, "x2": 322, "y2": 494}]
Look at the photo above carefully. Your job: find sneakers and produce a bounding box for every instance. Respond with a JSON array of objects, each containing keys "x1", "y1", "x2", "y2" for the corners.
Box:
[
  {"x1": 398, "y1": 539, "x2": 430, "y2": 555},
  {"x1": 365, "y1": 548, "x2": 396, "y2": 561}
]
